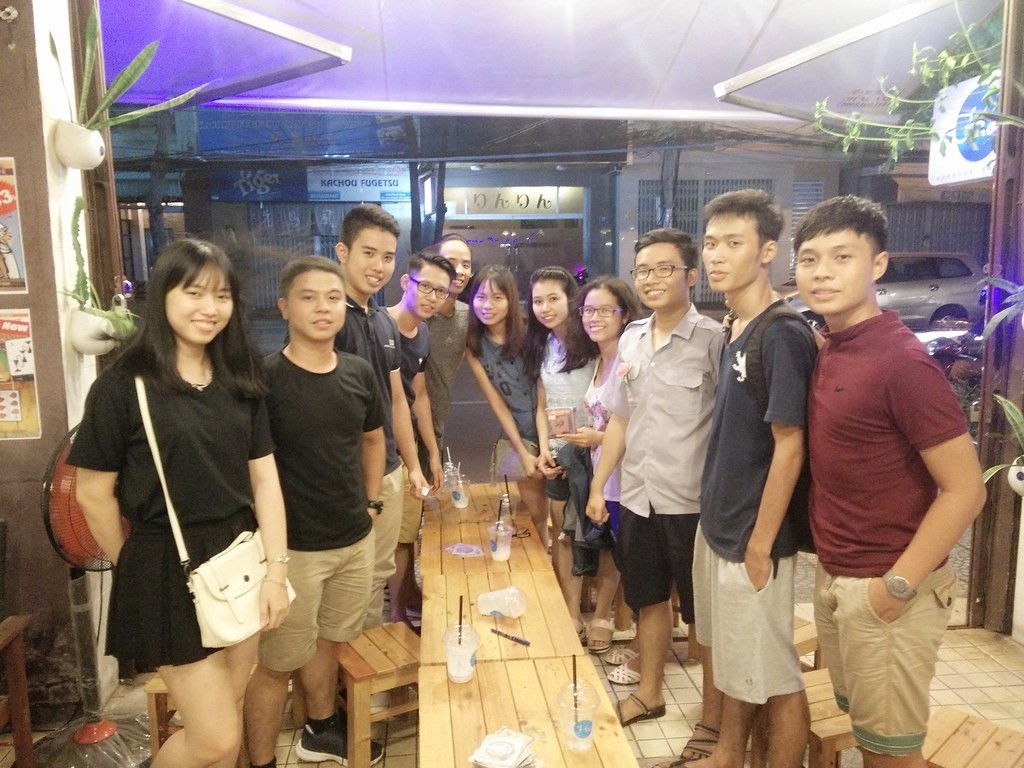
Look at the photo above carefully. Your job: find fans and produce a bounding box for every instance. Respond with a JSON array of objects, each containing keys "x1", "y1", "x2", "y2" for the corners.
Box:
[{"x1": 12, "y1": 421, "x2": 154, "y2": 768}]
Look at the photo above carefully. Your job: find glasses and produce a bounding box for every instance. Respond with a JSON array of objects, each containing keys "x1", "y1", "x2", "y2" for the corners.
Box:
[
  {"x1": 630, "y1": 265, "x2": 688, "y2": 280},
  {"x1": 409, "y1": 277, "x2": 450, "y2": 299},
  {"x1": 512, "y1": 528, "x2": 531, "y2": 537},
  {"x1": 579, "y1": 306, "x2": 625, "y2": 317}
]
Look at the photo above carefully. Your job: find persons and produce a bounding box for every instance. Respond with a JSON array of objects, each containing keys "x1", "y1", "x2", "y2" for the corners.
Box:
[
  {"x1": 723, "y1": 197, "x2": 987, "y2": 768},
  {"x1": 651, "y1": 189, "x2": 819, "y2": 768},
  {"x1": 244, "y1": 255, "x2": 387, "y2": 768},
  {"x1": 63, "y1": 240, "x2": 293, "y2": 768},
  {"x1": 285, "y1": 199, "x2": 643, "y2": 655},
  {"x1": 586, "y1": 228, "x2": 724, "y2": 761}
]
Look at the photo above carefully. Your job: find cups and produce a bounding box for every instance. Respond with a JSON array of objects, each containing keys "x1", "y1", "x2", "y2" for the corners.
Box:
[
  {"x1": 488, "y1": 523, "x2": 515, "y2": 561},
  {"x1": 450, "y1": 473, "x2": 471, "y2": 509},
  {"x1": 442, "y1": 622, "x2": 480, "y2": 684},
  {"x1": 496, "y1": 492, "x2": 519, "y2": 526},
  {"x1": 442, "y1": 462, "x2": 455, "y2": 489},
  {"x1": 476, "y1": 585, "x2": 527, "y2": 620},
  {"x1": 557, "y1": 677, "x2": 600, "y2": 754}
]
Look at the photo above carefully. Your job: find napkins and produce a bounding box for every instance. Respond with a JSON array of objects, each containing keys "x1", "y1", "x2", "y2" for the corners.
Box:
[{"x1": 466, "y1": 727, "x2": 538, "y2": 768}]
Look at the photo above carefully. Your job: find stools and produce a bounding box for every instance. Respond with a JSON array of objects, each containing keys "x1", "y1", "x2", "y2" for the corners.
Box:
[
  {"x1": 792, "y1": 617, "x2": 861, "y2": 768},
  {"x1": 336, "y1": 621, "x2": 422, "y2": 768},
  {"x1": 922, "y1": 705, "x2": 1024, "y2": 768}
]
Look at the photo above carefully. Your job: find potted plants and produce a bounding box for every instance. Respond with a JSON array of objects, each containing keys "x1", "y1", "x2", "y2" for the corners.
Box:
[
  {"x1": 980, "y1": 393, "x2": 1024, "y2": 496},
  {"x1": 60, "y1": 195, "x2": 140, "y2": 355},
  {"x1": 48, "y1": 1, "x2": 221, "y2": 169}
]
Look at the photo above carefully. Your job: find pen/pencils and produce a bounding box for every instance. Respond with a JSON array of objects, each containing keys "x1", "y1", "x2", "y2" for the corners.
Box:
[{"x1": 491, "y1": 627, "x2": 531, "y2": 646}]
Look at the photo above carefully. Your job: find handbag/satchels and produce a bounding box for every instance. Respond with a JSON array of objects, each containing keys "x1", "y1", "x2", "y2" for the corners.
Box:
[{"x1": 185, "y1": 528, "x2": 297, "y2": 649}]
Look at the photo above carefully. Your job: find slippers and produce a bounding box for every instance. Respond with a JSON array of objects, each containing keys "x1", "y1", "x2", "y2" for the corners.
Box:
[
  {"x1": 680, "y1": 724, "x2": 721, "y2": 762},
  {"x1": 616, "y1": 694, "x2": 666, "y2": 727}
]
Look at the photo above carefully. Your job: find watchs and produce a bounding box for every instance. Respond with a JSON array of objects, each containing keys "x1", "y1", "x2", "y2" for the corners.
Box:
[
  {"x1": 883, "y1": 570, "x2": 917, "y2": 601},
  {"x1": 368, "y1": 499, "x2": 383, "y2": 515},
  {"x1": 267, "y1": 553, "x2": 290, "y2": 566}
]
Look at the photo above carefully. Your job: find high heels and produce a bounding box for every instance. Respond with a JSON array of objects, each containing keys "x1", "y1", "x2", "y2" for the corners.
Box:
[{"x1": 587, "y1": 620, "x2": 614, "y2": 654}]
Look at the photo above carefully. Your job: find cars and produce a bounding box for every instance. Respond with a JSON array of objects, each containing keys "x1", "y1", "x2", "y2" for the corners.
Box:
[{"x1": 772, "y1": 252, "x2": 988, "y2": 332}]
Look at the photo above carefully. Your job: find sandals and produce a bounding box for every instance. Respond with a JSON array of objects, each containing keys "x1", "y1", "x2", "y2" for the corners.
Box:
[
  {"x1": 607, "y1": 663, "x2": 641, "y2": 684},
  {"x1": 604, "y1": 645, "x2": 638, "y2": 664}
]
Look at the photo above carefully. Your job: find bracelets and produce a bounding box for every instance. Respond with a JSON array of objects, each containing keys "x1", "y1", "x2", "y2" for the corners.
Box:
[{"x1": 263, "y1": 578, "x2": 288, "y2": 589}]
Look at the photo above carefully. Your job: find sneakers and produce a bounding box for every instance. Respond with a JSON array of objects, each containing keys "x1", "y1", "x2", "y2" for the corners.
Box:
[{"x1": 295, "y1": 719, "x2": 384, "y2": 767}]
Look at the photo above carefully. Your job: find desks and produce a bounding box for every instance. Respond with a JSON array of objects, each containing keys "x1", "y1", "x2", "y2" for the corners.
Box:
[{"x1": 420, "y1": 481, "x2": 640, "y2": 767}]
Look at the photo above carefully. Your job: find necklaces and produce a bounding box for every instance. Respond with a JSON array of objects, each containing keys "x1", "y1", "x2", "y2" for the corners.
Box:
[{"x1": 191, "y1": 383, "x2": 208, "y2": 388}]
